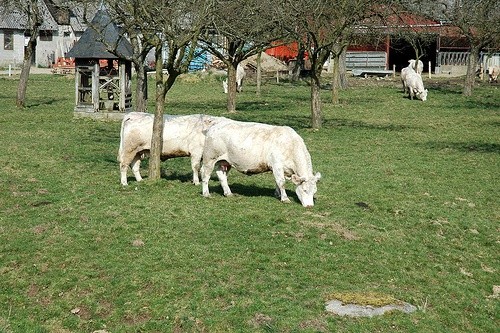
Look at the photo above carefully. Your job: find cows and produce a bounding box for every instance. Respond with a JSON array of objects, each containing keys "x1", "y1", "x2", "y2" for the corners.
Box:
[
  {"x1": 200, "y1": 116, "x2": 322, "y2": 208},
  {"x1": 400, "y1": 67, "x2": 428, "y2": 101},
  {"x1": 488, "y1": 64, "x2": 500, "y2": 84},
  {"x1": 408, "y1": 59, "x2": 423, "y2": 75},
  {"x1": 117, "y1": 111, "x2": 216, "y2": 186},
  {"x1": 223, "y1": 63, "x2": 247, "y2": 94}
]
